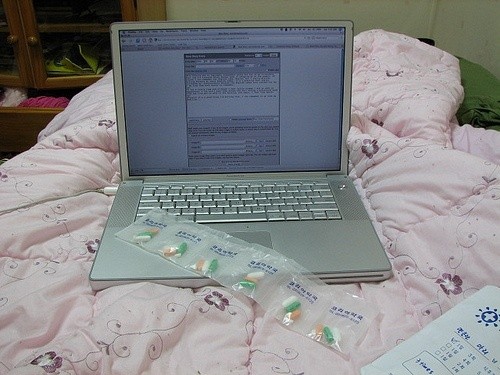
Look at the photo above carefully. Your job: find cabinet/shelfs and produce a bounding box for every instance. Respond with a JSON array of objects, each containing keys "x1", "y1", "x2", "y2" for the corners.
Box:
[{"x1": 0, "y1": 0, "x2": 168, "y2": 158}]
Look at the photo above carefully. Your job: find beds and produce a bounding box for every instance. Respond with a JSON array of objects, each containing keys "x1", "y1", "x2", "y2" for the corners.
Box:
[{"x1": 0, "y1": 29, "x2": 500, "y2": 375}]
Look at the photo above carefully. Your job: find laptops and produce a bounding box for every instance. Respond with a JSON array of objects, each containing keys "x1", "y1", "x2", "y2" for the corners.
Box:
[{"x1": 89, "y1": 20, "x2": 393, "y2": 291}]
